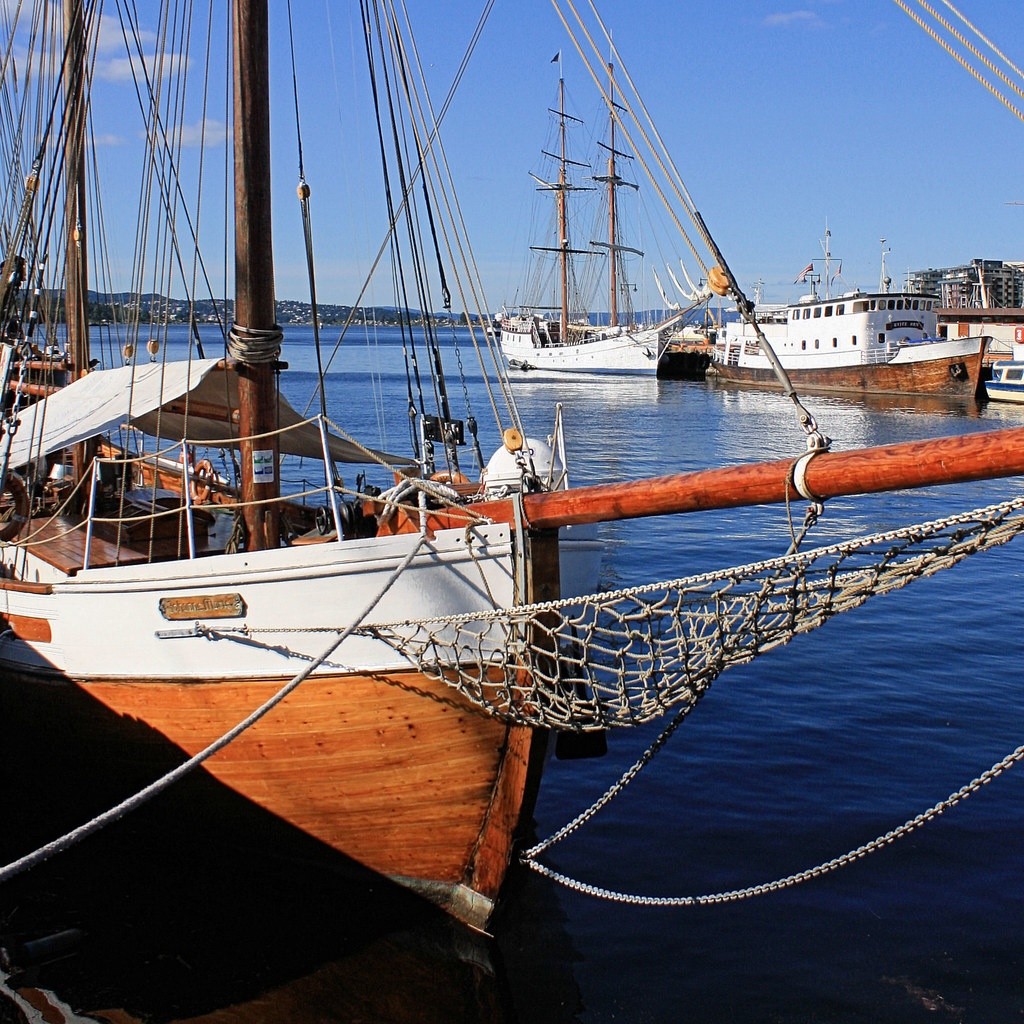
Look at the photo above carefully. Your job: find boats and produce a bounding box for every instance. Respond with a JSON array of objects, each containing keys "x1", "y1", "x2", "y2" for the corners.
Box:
[
  {"x1": 704, "y1": 222, "x2": 992, "y2": 400},
  {"x1": 984, "y1": 343, "x2": 1024, "y2": 405}
]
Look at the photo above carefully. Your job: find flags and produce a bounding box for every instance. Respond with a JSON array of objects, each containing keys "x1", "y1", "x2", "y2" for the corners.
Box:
[
  {"x1": 793, "y1": 263, "x2": 813, "y2": 284},
  {"x1": 551, "y1": 52, "x2": 559, "y2": 63}
]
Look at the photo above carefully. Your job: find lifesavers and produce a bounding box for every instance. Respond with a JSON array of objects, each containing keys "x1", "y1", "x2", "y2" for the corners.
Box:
[
  {"x1": 0, "y1": 467, "x2": 33, "y2": 541},
  {"x1": 190, "y1": 460, "x2": 214, "y2": 504},
  {"x1": 431, "y1": 470, "x2": 470, "y2": 486}
]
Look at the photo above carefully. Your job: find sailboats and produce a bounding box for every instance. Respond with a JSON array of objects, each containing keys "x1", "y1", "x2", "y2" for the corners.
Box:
[
  {"x1": 0, "y1": 0, "x2": 1024, "y2": 952},
  {"x1": 496, "y1": 33, "x2": 714, "y2": 379}
]
